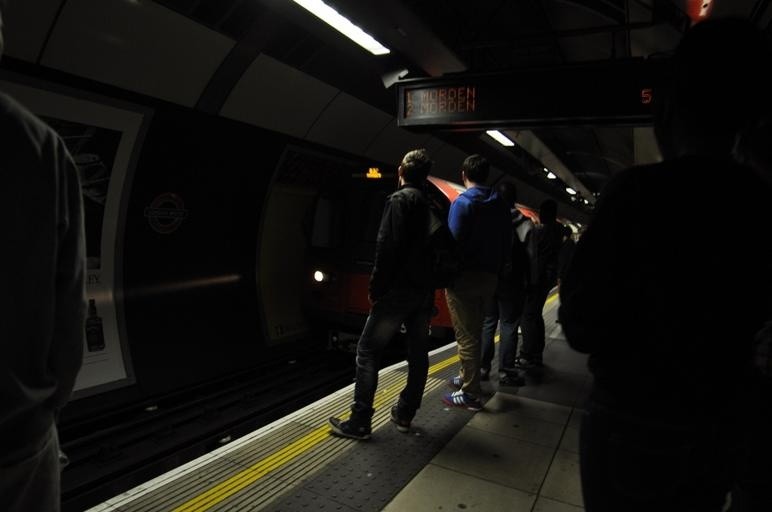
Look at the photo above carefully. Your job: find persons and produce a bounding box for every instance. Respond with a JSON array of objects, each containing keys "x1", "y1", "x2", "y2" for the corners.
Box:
[
  {"x1": 1, "y1": 90, "x2": 88, "y2": 512},
  {"x1": 556, "y1": 91, "x2": 772, "y2": 511},
  {"x1": 329, "y1": 147, "x2": 448, "y2": 440},
  {"x1": 443, "y1": 152, "x2": 576, "y2": 412}
]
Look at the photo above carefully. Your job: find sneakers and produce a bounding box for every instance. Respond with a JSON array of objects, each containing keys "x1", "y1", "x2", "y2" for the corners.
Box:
[
  {"x1": 391, "y1": 405, "x2": 412, "y2": 431},
  {"x1": 328, "y1": 416, "x2": 371, "y2": 439},
  {"x1": 441, "y1": 376, "x2": 482, "y2": 410},
  {"x1": 514, "y1": 355, "x2": 543, "y2": 368},
  {"x1": 499, "y1": 374, "x2": 525, "y2": 386}
]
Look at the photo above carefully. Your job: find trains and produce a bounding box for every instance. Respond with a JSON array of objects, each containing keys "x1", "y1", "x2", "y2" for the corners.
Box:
[{"x1": 300, "y1": 164, "x2": 584, "y2": 350}]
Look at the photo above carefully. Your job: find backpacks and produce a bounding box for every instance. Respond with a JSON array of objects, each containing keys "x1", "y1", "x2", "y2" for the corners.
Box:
[
  {"x1": 458, "y1": 191, "x2": 505, "y2": 269},
  {"x1": 501, "y1": 229, "x2": 524, "y2": 283}
]
